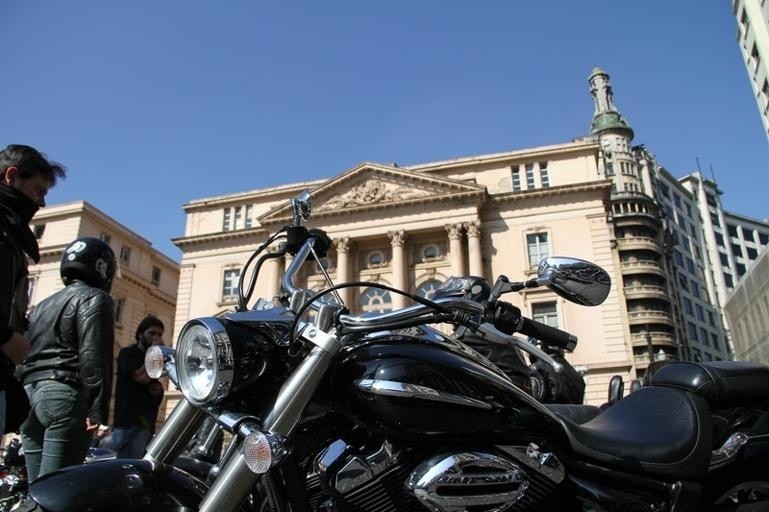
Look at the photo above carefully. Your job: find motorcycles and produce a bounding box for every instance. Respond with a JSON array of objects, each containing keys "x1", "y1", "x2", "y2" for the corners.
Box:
[
  {"x1": 0, "y1": 433, "x2": 119, "y2": 512},
  {"x1": 22, "y1": 187, "x2": 769, "y2": 512}
]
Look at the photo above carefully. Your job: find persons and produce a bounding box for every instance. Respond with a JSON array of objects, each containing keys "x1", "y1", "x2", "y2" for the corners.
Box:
[
  {"x1": 20, "y1": 237, "x2": 118, "y2": 486},
  {"x1": 1, "y1": 144, "x2": 67, "y2": 391},
  {"x1": 97, "y1": 316, "x2": 165, "y2": 459}
]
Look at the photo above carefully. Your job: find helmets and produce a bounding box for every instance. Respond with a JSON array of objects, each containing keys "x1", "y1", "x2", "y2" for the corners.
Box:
[{"x1": 57, "y1": 234, "x2": 119, "y2": 291}]
